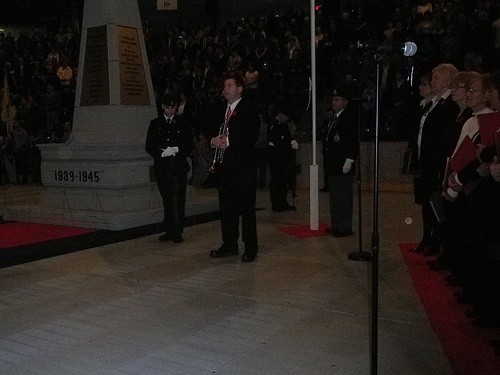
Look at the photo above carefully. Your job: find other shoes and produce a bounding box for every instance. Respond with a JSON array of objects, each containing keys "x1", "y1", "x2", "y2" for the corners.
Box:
[
  {"x1": 285, "y1": 205, "x2": 296, "y2": 210},
  {"x1": 272, "y1": 208, "x2": 283, "y2": 211},
  {"x1": 333, "y1": 231, "x2": 352, "y2": 237},
  {"x1": 325, "y1": 227, "x2": 338, "y2": 233},
  {"x1": 410, "y1": 238, "x2": 500, "y2": 329}
]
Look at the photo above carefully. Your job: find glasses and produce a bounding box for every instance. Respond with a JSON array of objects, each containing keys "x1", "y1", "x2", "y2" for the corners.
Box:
[
  {"x1": 469, "y1": 87, "x2": 481, "y2": 93},
  {"x1": 449, "y1": 85, "x2": 466, "y2": 90}
]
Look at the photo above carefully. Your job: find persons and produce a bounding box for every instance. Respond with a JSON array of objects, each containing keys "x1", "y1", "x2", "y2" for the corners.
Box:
[
  {"x1": 143, "y1": 93, "x2": 190, "y2": 243},
  {"x1": 266, "y1": 106, "x2": 298, "y2": 211},
  {"x1": 322, "y1": 87, "x2": 360, "y2": 237},
  {"x1": 0, "y1": 0, "x2": 500, "y2": 354},
  {"x1": 209, "y1": 75, "x2": 260, "y2": 262}
]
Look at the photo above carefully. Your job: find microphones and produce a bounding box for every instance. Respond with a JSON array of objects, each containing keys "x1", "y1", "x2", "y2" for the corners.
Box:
[{"x1": 358, "y1": 40, "x2": 418, "y2": 56}]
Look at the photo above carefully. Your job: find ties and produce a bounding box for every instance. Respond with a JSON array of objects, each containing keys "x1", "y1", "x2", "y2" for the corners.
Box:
[
  {"x1": 167, "y1": 119, "x2": 171, "y2": 130},
  {"x1": 225, "y1": 107, "x2": 231, "y2": 129}
]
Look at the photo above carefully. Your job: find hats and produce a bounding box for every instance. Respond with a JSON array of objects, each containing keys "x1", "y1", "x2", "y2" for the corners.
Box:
[
  {"x1": 160, "y1": 94, "x2": 176, "y2": 106},
  {"x1": 332, "y1": 88, "x2": 343, "y2": 97}
]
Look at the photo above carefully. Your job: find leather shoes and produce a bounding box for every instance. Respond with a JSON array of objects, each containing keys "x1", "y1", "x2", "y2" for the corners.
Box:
[
  {"x1": 210, "y1": 246, "x2": 238, "y2": 257},
  {"x1": 242, "y1": 247, "x2": 257, "y2": 262},
  {"x1": 173, "y1": 231, "x2": 182, "y2": 243},
  {"x1": 159, "y1": 232, "x2": 173, "y2": 241}
]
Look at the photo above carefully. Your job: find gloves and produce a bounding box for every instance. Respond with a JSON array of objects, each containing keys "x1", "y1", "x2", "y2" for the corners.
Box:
[
  {"x1": 342, "y1": 158, "x2": 354, "y2": 174},
  {"x1": 161, "y1": 146, "x2": 179, "y2": 158},
  {"x1": 291, "y1": 139, "x2": 298, "y2": 150}
]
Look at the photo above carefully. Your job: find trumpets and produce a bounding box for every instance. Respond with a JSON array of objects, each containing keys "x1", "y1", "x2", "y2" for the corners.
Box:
[{"x1": 205, "y1": 123, "x2": 229, "y2": 174}]
